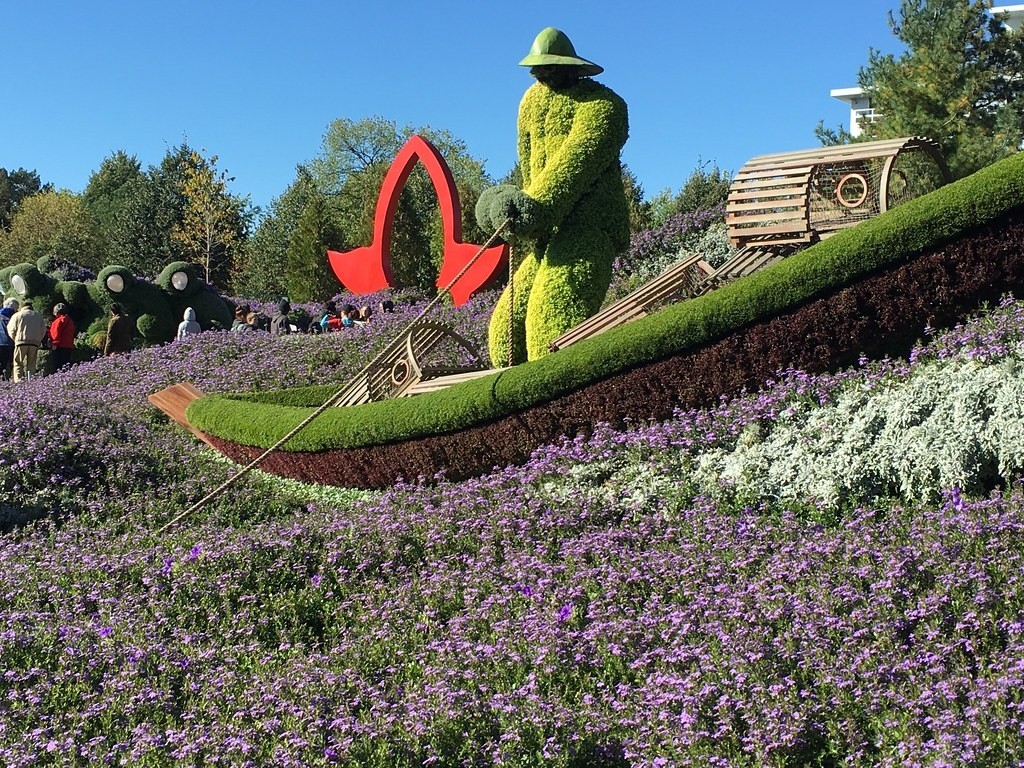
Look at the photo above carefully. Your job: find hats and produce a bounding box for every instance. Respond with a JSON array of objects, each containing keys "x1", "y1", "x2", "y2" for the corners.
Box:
[
  {"x1": 342, "y1": 304, "x2": 353, "y2": 312},
  {"x1": 53, "y1": 303, "x2": 65, "y2": 315},
  {"x1": 279, "y1": 301, "x2": 290, "y2": 311}
]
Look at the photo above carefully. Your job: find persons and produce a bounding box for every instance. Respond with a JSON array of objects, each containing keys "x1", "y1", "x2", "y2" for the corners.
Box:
[
  {"x1": 177, "y1": 307, "x2": 201, "y2": 342},
  {"x1": 103, "y1": 303, "x2": 133, "y2": 357},
  {"x1": 473, "y1": 25, "x2": 633, "y2": 372},
  {"x1": 226, "y1": 293, "x2": 392, "y2": 336},
  {"x1": 6, "y1": 300, "x2": 48, "y2": 384},
  {"x1": 47, "y1": 302, "x2": 74, "y2": 376},
  {"x1": 0, "y1": 297, "x2": 21, "y2": 381}
]
